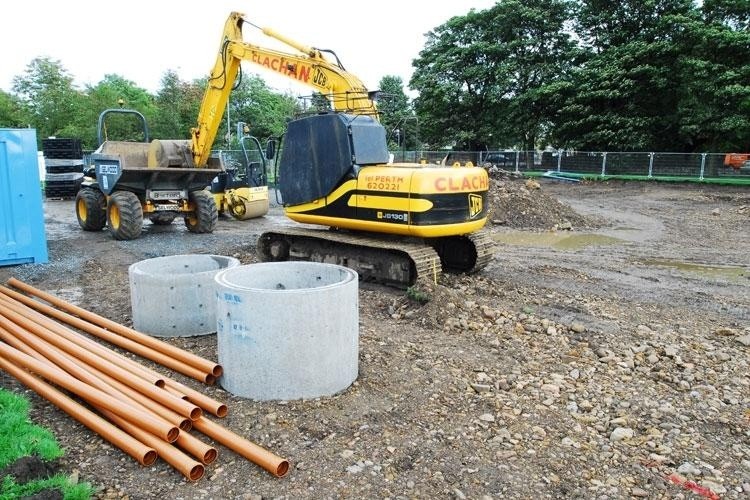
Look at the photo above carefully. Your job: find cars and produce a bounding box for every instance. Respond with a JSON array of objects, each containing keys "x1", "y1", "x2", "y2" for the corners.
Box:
[{"x1": 739, "y1": 160, "x2": 750, "y2": 176}]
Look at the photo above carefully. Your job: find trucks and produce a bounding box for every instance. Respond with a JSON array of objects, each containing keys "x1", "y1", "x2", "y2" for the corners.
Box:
[{"x1": 722, "y1": 153, "x2": 750, "y2": 172}]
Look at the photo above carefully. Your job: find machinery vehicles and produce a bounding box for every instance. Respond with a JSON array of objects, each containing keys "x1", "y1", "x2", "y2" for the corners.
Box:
[
  {"x1": 193, "y1": 9, "x2": 491, "y2": 286},
  {"x1": 73, "y1": 108, "x2": 217, "y2": 240},
  {"x1": 208, "y1": 119, "x2": 274, "y2": 220}
]
